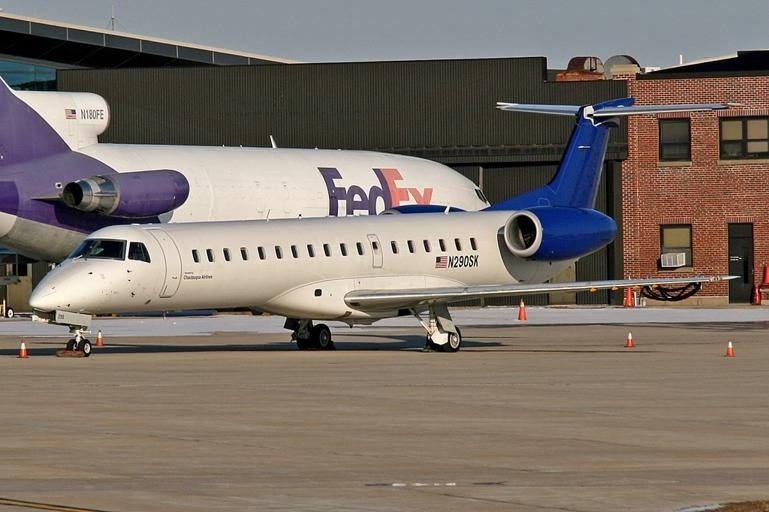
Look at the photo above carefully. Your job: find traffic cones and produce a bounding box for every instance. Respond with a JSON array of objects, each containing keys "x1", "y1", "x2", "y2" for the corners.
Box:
[
  {"x1": 750, "y1": 283, "x2": 760, "y2": 306},
  {"x1": 622, "y1": 285, "x2": 633, "y2": 308},
  {"x1": 760, "y1": 264, "x2": 769, "y2": 288},
  {"x1": 515, "y1": 298, "x2": 527, "y2": 321},
  {"x1": 17, "y1": 337, "x2": 29, "y2": 358},
  {"x1": 722, "y1": 339, "x2": 735, "y2": 357},
  {"x1": 94, "y1": 330, "x2": 104, "y2": 347},
  {"x1": 621, "y1": 330, "x2": 634, "y2": 347}
]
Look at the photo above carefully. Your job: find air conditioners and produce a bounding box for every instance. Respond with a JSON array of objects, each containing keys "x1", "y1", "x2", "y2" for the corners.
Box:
[{"x1": 660, "y1": 252, "x2": 686, "y2": 267}]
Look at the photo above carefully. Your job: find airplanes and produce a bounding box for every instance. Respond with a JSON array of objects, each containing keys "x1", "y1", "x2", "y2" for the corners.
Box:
[
  {"x1": 27, "y1": 94, "x2": 745, "y2": 354},
  {"x1": 0, "y1": 73, "x2": 492, "y2": 262}
]
